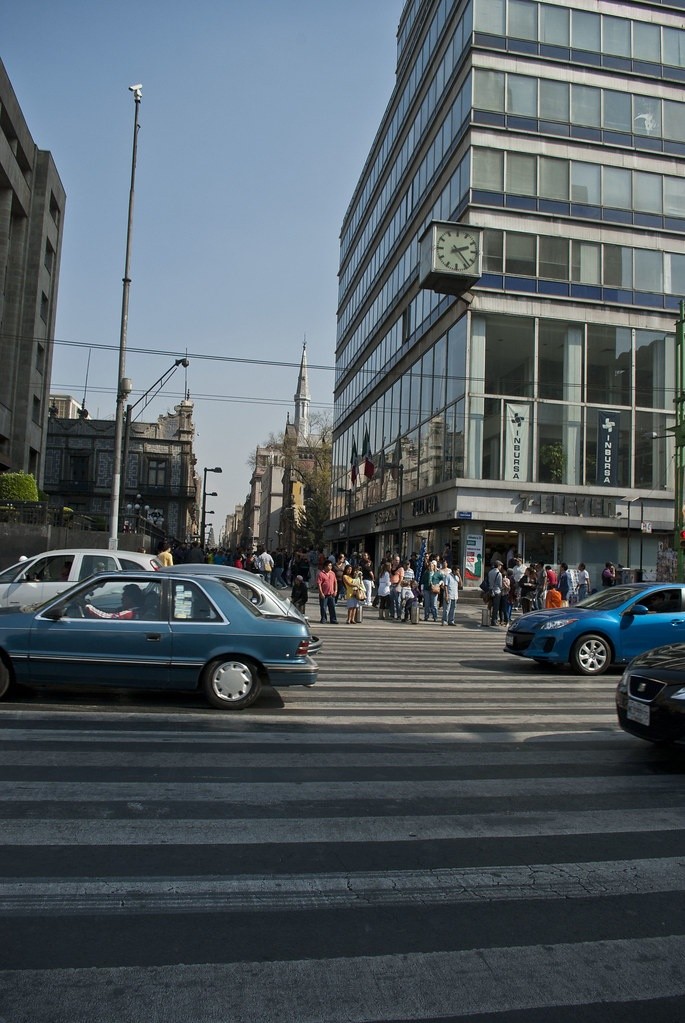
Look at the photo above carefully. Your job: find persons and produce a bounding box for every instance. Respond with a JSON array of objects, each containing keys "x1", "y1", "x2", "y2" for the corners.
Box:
[
  {"x1": 307, "y1": 546, "x2": 463, "y2": 626},
  {"x1": 157, "y1": 545, "x2": 173, "y2": 567},
  {"x1": 172, "y1": 541, "x2": 289, "y2": 590},
  {"x1": 34, "y1": 561, "x2": 73, "y2": 582},
  {"x1": 480, "y1": 547, "x2": 570, "y2": 626},
  {"x1": 290, "y1": 575, "x2": 308, "y2": 611},
  {"x1": 85, "y1": 584, "x2": 144, "y2": 620},
  {"x1": 575, "y1": 562, "x2": 590, "y2": 601},
  {"x1": 602, "y1": 561, "x2": 615, "y2": 587},
  {"x1": 317, "y1": 560, "x2": 339, "y2": 624},
  {"x1": 137, "y1": 547, "x2": 147, "y2": 554}
]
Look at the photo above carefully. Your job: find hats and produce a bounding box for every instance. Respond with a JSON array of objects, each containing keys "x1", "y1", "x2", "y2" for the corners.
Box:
[
  {"x1": 97, "y1": 562, "x2": 106, "y2": 568},
  {"x1": 494, "y1": 560, "x2": 503, "y2": 566},
  {"x1": 400, "y1": 580, "x2": 408, "y2": 587},
  {"x1": 19, "y1": 556, "x2": 28, "y2": 562},
  {"x1": 297, "y1": 575, "x2": 304, "y2": 581}
]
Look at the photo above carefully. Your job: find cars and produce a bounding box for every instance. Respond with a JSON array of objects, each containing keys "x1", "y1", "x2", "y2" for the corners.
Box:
[
  {"x1": 502, "y1": 580, "x2": 685, "y2": 677},
  {"x1": 0, "y1": 547, "x2": 165, "y2": 609},
  {"x1": 615, "y1": 641, "x2": 685, "y2": 755},
  {"x1": 0, "y1": 571, "x2": 321, "y2": 712},
  {"x1": 87, "y1": 562, "x2": 324, "y2": 657}
]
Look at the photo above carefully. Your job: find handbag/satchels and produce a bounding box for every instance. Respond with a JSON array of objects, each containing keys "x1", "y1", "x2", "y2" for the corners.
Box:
[
  {"x1": 479, "y1": 580, "x2": 489, "y2": 590},
  {"x1": 264, "y1": 564, "x2": 272, "y2": 572},
  {"x1": 353, "y1": 588, "x2": 365, "y2": 601},
  {"x1": 481, "y1": 590, "x2": 494, "y2": 603},
  {"x1": 431, "y1": 585, "x2": 440, "y2": 594}
]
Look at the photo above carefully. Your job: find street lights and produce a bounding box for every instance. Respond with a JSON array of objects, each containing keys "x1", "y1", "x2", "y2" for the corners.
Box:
[
  {"x1": 108, "y1": 357, "x2": 190, "y2": 550},
  {"x1": 200, "y1": 467, "x2": 222, "y2": 549}
]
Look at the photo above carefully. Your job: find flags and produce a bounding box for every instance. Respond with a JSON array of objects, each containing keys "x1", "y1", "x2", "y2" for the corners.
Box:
[
  {"x1": 362, "y1": 423, "x2": 375, "y2": 480},
  {"x1": 391, "y1": 425, "x2": 403, "y2": 480},
  {"x1": 350, "y1": 434, "x2": 359, "y2": 487},
  {"x1": 378, "y1": 436, "x2": 386, "y2": 485}
]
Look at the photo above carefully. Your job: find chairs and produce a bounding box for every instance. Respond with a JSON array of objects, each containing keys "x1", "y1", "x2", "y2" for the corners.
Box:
[{"x1": 143, "y1": 591, "x2": 160, "y2": 620}]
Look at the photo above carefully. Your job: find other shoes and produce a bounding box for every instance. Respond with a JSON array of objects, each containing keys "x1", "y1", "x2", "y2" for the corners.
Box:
[
  {"x1": 281, "y1": 585, "x2": 288, "y2": 590},
  {"x1": 448, "y1": 623, "x2": 456, "y2": 626},
  {"x1": 367, "y1": 603, "x2": 372, "y2": 606},
  {"x1": 442, "y1": 622, "x2": 448, "y2": 626},
  {"x1": 346, "y1": 620, "x2": 350, "y2": 624},
  {"x1": 372, "y1": 605, "x2": 378, "y2": 608},
  {"x1": 351, "y1": 620, "x2": 356, "y2": 623},
  {"x1": 330, "y1": 620, "x2": 338, "y2": 624},
  {"x1": 320, "y1": 620, "x2": 325, "y2": 624}
]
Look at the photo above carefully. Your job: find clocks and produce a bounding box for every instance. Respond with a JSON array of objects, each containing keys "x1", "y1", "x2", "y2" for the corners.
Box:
[
  {"x1": 438, "y1": 230, "x2": 478, "y2": 270},
  {"x1": 420, "y1": 235, "x2": 431, "y2": 279}
]
